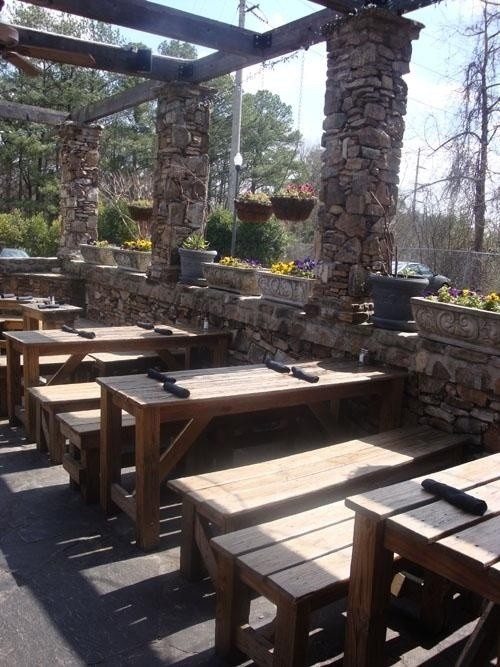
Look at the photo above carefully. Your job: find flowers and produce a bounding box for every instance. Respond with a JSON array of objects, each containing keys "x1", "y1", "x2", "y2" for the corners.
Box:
[
  {"x1": 219, "y1": 256, "x2": 261, "y2": 269},
  {"x1": 235, "y1": 192, "x2": 273, "y2": 206},
  {"x1": 120, "y1": 240, "x2": 152, "y2": 251},
  {"x1": 86, "y1": 239, "x2": 109, "y2": 248},
  {"x1": 271, "y1": 256, "x2": 320, "y2": 277},
  {"x1": 421, "y1": 284, "x2": 500, "y2": 313},
  {"x1": 287, "y1": 183, "x2": 316, "y2": 199}
]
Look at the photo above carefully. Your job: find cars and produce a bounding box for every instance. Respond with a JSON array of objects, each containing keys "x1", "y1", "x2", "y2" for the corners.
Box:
[
  {"x1": 0, "y1": 248, "x2": 29, "y2": 259},
  {"x1": 392, "y1": 261, "x2": 452, "y2": 291}
]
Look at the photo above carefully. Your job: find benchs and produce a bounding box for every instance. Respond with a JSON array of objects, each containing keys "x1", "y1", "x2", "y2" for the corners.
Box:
[{"x1": 167, "y1": 421, "x2": 473, "y2": 580}]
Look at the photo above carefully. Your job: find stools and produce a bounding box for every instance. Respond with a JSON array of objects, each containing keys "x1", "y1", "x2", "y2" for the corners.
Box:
[
  {"x1": 26, "y1": 382, "x2": 101, "y2": 458},
  {"x1": 55, "y1": 409, "x2": 136, "y2": 505},
  {"x1": 0, "y1": 318, "x2": 24, "y2": 348},
  {"x1": 88, "y1": 346, "x2": 190, "y2": 377},
  {"x1": 209, "y1": 500, "x2": 422, "y2": 667},
  {"x1": 0, "y1": 354, "x2": 96, "y2": 417}
]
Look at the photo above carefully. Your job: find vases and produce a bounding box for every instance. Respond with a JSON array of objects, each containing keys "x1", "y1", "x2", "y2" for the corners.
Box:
[
  {"x1": 201, "y1": 262, "x2": 262, "y2": 296},
  {"x1": 80, "y1": 244, "x2": 116, "y2": 265},
  {"x1": 235, "y1": 204, "x2": 274, "y2": 222},
  {"x1": 111, "y1": 247, "x2": 152, "y2": 273},
  {"x1": 256, "y1": 271, "x2": 317, "y2": 307},
  {"x1": 269, "y1": 197, "x2": 317, "y2": 221},
  {"x1": 409, "y1": 297, "x2": 500, "y2": 356}
]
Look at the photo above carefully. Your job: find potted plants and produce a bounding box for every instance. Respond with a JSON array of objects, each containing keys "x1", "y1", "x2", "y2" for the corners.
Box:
[
  {"x1": 178, "y1": 231, "x2": 217, "y2": 281},
  {"x1": 368, "y1": 272, "x2": 429, "y2": 330},
  {"x1": 128, "y1": 199, "x2": 153, "y2": 222}
]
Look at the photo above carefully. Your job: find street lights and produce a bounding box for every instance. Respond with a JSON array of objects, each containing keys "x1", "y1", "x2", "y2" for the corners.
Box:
[{"x1": 230, "y1": 153, "x2": 243, "y2": 256}]
